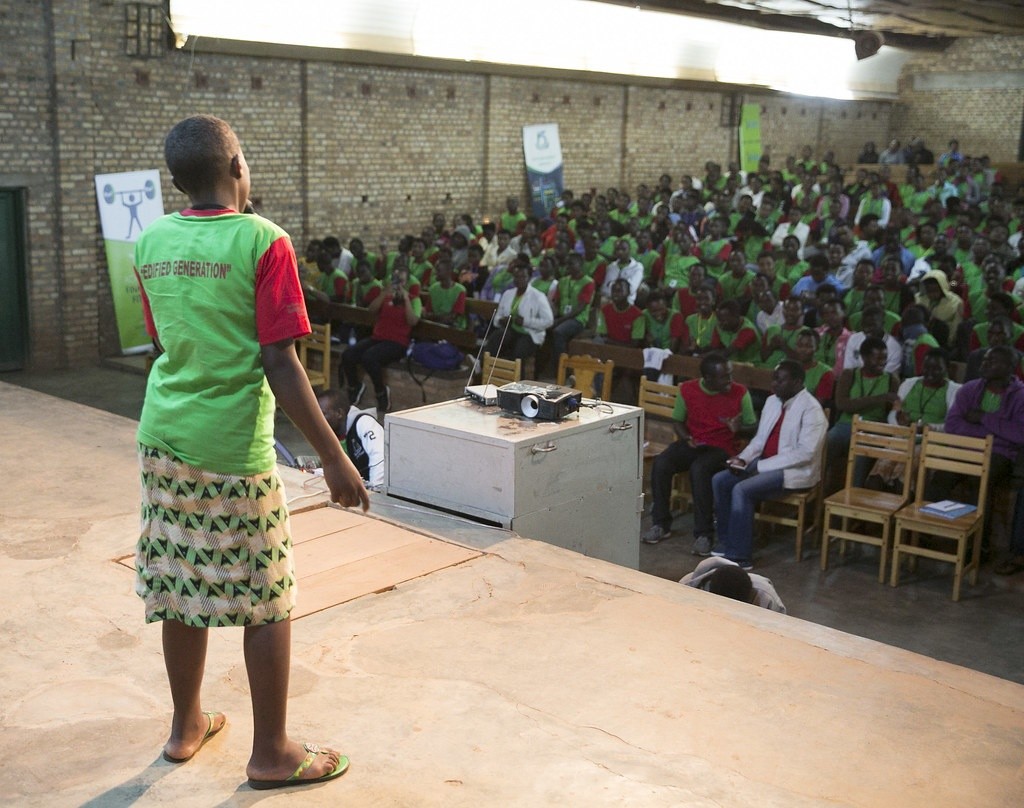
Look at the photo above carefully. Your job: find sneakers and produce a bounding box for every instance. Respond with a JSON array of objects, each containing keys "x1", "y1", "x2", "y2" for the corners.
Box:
[
  {"x1": 727, "y1": 559, "x2": 753, "y2": 571},
  {"x1": 375, "y1": 385, "x2": 390, "y2": 412},
  {"x1": 642, "y1": 525, "x2": 671, "y2": 545},
  {"x1": 691, "y1": 536, "x2": 713, "y2": 556},
  {"x1": 348, "y1": 382, "x2": 365, "y2": 406},
  {"x1": 711, "y1": 541, "x2": 728, "y2": 558}
]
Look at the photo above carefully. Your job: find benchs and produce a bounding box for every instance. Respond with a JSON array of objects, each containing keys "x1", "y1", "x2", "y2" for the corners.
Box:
[{"x1": 301, "y1": 283, "x2": 775, "y2": 406}]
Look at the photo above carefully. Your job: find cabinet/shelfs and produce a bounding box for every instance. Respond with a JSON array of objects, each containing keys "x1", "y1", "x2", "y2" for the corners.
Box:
[{"x1": 381, "y1": 400, "x2": 646, "y2": 572}]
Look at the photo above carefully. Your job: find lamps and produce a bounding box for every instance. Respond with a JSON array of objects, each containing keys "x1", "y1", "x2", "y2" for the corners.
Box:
[{"x1": 847, "y1": 0, "x2": 888, "y2": 62}]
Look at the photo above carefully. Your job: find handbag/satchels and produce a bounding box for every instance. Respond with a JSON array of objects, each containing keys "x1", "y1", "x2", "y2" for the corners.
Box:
[{"x1": 407, "y1": 339, "x2": 465, "y2": 386}]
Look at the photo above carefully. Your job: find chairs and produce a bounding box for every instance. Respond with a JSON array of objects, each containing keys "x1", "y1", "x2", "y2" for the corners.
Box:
[
  {"x1": 752, "y1": 408, "x2": 832, "y2": 564},
  {"x1": 633, "y1": 371, "x2": 684, "y2": 509},
  {"x1": 889, "y1": 424, "x2": 997, "y2": 604},
  {"x1": 276, "y1": 321, "x2": 335, "y2": 407},
  {"x1": 556, "y1": 350, "x2": 615, "y2": 401},
  {"x1": 481, "y1": 350, "x2": 524, "y2": 389},
  {"x1": 816, "y1": 411, "x2": 917, "y2": 585}
]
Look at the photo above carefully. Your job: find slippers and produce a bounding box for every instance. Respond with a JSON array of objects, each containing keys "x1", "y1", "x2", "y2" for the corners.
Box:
[
  {"x1": 248, "y1": 743, "x2": 350, "y2": 790},
  {"x1": 163, "y1": 711, "x2": 226, "y2": 763}
]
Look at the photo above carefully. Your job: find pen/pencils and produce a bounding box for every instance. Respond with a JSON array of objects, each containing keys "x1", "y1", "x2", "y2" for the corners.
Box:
[{"x1": 944, "y1": 502, "x2": 958, "y2": 508}]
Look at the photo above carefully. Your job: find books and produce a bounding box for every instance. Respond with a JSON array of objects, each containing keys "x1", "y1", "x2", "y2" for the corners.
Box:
[{"x1": 919, "y1": 499, "x2": 977, "y2": 519}]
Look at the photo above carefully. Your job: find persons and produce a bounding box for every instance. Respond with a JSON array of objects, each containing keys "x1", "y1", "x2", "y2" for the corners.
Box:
[
  {"x1": 287, "y1": 136, "x2": 1024, "y2": 568},
  {"x1": 132, "y1": 115, "x2": 372, "y2": 790},
  {"x1": 679, "y1": 556, "x2": 787, "y2": 615}
]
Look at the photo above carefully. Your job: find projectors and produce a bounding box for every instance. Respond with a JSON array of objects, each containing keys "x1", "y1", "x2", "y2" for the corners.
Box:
[{"x1": 495, "y1": 380, "x2": 582, "y2": 419}]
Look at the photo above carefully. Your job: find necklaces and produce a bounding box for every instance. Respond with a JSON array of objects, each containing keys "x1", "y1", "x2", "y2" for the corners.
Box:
[
  {"x1": 697, "y1": 312, "x2": 713, "y2": 345},
  {"x1": 857, "y1": 370, "x2": 880, "y2": 414},
  {"x1": 823, "y1": 332, "x2": 834, "y2": 362},
  {"x1": 919, "y1": 383, "x2": 942, "y2": 423},
  {"x1": 886, "y1": 291, "x2": 897, "y2": 310},
  {"x1": 193, "y1": 204, "x2": 228, "y2": 210}
]
